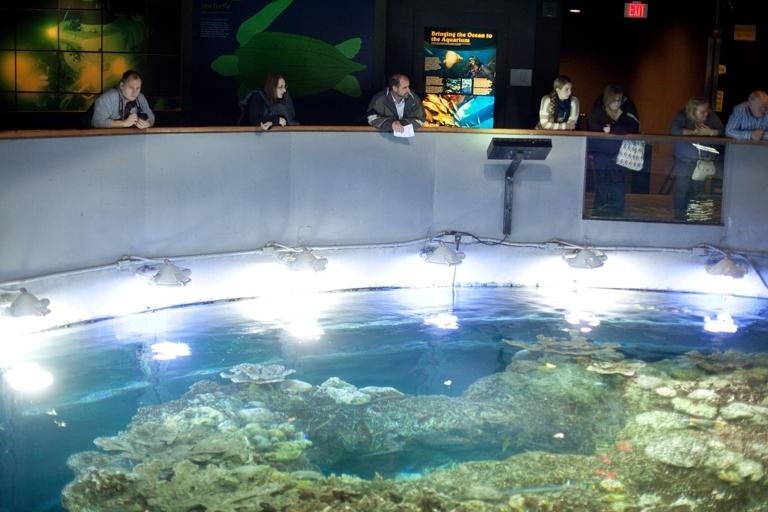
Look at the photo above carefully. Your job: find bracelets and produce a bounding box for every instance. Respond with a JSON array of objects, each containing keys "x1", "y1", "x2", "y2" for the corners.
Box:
[{"x1": 710, "y1": 129, "x2": 715, "y2": 136}]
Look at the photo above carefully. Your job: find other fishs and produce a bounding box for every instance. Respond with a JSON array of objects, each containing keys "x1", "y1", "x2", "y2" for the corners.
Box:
[
  {"x1": 690, "y1": 418, "x2": 726, "y2": 429},
  {"x1": 422, "y1": 93, "x2": 461, "y2": 128},
  {"x1": 502, "y1": 336, "x2": 534, "y2": 349},
  {"x1": 443, "y1": 50, "x2": 463, "y2": 69}
]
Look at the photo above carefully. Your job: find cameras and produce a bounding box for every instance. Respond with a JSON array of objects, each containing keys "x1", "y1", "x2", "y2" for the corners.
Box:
[{"x1": 136, "y1": 106, "x2": 148, "y2": 121}]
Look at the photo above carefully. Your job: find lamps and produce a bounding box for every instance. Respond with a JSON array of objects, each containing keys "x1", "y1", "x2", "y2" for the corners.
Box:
[
  {"x1": 255, "y1": 241, "x2": 328, "y2": 274},
  {"x1": 1, "y1": 283, "x2": 55, "y2": 318},
  {"x1": 417, "y1": 234, "x2": 466, "y2": 266},
  {"x1": 694, "y1": 242, "x2": 748, "y2": 280},
  {"x1": 544, "y1": 238, "x2": 608, "y2": 270},
  {"x1": 116, "y1": 253, "x2": 193, "y2": 287}
]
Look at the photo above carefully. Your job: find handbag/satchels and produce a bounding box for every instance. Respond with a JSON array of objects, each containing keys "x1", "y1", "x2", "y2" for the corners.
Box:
[
  {"x1": 616, "y1": 140, "x2": 646, "y2": 171},
  {"x1": 691, "y1": 160, "x2": 716, "y2": 181}
]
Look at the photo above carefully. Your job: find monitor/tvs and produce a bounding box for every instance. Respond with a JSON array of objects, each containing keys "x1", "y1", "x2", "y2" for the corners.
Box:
[{"x1": 488, "y1": 138, "x2": 552, "y2": 160}]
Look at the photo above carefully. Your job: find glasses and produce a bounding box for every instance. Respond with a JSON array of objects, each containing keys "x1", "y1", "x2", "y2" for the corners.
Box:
[{"x1": 276, "y1": 84, "x2": 288, "y2": 89}]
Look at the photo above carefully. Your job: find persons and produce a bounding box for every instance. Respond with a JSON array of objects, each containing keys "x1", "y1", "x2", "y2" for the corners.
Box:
[
  {"x1": 91, "y1": 69, "x2": 155, "y2": 130},
  {"x1": 725, "y1": 89, "x2": 768, "y2": 141},
  {"x1": 666, "y1": 94, "x2": 723, "y2": 221},
  {"x1": 588, "y1": 84, "x2": 641, "y2": 217},
  {"x1": 535, "y1": 74, "x2": 581, "y2": 130},
  {"x1": 239, "y1": 73, "x2": 298, "y2": 131},
  {"x1": 362, "y1": 74, "x2": 427, "y2": 133}
]
